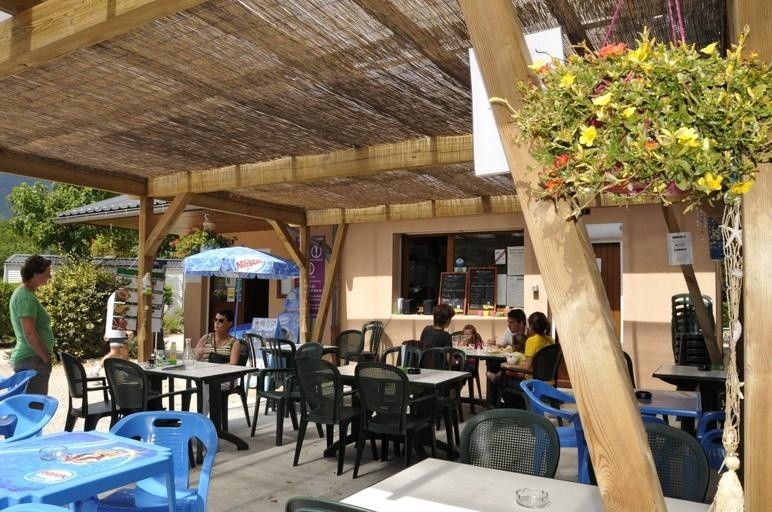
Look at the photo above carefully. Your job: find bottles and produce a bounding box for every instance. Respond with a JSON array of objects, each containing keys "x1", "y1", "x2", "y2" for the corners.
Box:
[
  {"x1": 183, "y1": 338, "x2": 197, "y2": 370},
  {"x1": 150, "y1": 350, "x2": 164, "y2": 368}
]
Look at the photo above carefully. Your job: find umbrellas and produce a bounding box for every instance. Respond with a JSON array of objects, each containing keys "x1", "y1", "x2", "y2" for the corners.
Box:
[{"x1": 183, "y1": 246, "x2": 299, "y2": 338}]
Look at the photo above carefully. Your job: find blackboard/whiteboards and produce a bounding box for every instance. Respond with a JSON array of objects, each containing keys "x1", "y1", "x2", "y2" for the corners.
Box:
[
  {"x1": 438, "y1": 272, "x2": 468, "y2": 315},
  {"x1": 467, "y1": 266, "x2": 497, "y2": 314}
]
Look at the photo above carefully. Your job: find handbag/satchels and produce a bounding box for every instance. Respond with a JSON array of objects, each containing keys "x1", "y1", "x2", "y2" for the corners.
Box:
[{"x1": 207, "y1": 352, "x2": 230, "y2": 364}]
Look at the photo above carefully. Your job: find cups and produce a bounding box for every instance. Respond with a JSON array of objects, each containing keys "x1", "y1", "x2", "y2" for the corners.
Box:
[{"x1": 452, "y1": 335, "x2": 474, "y2": 351}]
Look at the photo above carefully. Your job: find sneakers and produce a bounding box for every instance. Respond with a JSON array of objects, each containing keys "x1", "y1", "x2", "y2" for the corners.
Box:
[
  {"x1": 487, "y1": 371, "x2": 496, "y2": 383},
  {"x1": 479, "y1": 404, "x2": 495, "y2": 412}
]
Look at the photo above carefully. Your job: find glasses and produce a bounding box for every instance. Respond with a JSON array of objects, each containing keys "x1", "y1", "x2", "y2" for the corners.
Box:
[{"x1": 213, "y1": 318, "x2": 224, "y2": 323}]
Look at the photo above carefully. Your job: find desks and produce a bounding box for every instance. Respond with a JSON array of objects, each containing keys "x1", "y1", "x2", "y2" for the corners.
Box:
[
  {"x1": 634, "y1": 385, "x2": 703, "y2": 431},
  {"x1": 132, "y1": 358, "x2": 260, "y2": 466},
  {"x1": 338, "y1": 456, "x2": 709, "y2": 511},
  {"x1": 0, "y1": 428, "x2": 177, "y2": 512},
  {"x1": 652, "y1": 363, "x2": 728, "y2": 448}
]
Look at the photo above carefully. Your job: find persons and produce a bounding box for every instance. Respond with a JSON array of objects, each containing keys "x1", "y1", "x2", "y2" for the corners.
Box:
[
  {"x1": 86, "y1": 288, "x2": 134, "y2": 384},
  {"x1": 487, "y1": 312, "x2": 557, "y2": 409},
  {"x1": 9, "y1": 255, "x2": 52, "y2": 408},
  {"x1": 487, "y1": 334, "x2": 528, "y2": 382},
  {"x1": 194, "y1": 308, "x2": 241, "y2": 416},
  {"x1": 488, "y1": 308, "x2": 529, "y2": 349},
  {"x1": 421, "y1": 304, "x2": 465, "y2": 403},
  {"x1": 455, "y1": 325, "x2": 481, "y2": 377}
]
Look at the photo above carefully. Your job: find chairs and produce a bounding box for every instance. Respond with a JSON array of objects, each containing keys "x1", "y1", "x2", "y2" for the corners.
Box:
[
  {"x1": 516, "y1": 377, "x2": 595, "y2": 487},
  {"x1": 670, "y1": 292, "x2": 715, "y2": 422},
  {"x1": 221, "y1": 339, "x2": 251, "y2": 433},
  {"x1": 58, "y1": 351, "x2": 123, "y2": 432},
  {"x1": 0, "y1": 392, "x2": 59, "y2": 444},
  {"x1": 640, "y1": 413, "x2": 710, "y2": 506},
  {"x1": 0, "y1": 368, "x2": 38, "y2": 440},
  {"x1": 67, "y1": 410, "x2": 219, "y2": 512},
  {"x1": 694, "y1": 408, "x2": 731, "y2": 471},
  {"x1": 103, "y1": 357, "x2": 197, "y2": 469},
  {"x1": 285, "y1": 495, "x2": 375, "y2": 512}
]
